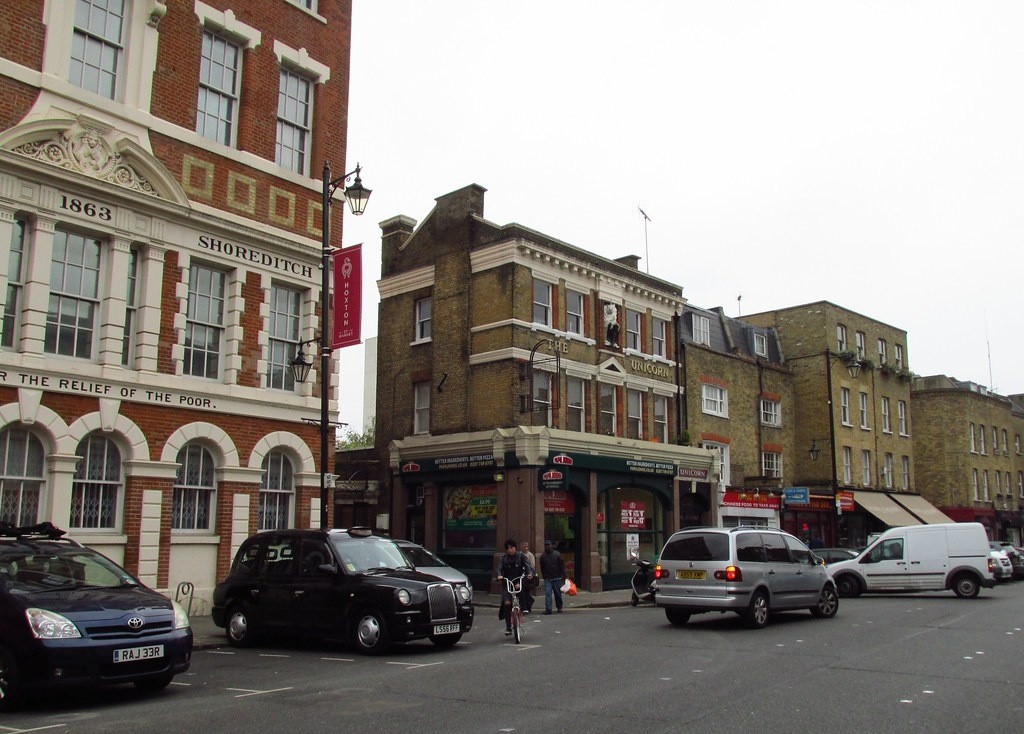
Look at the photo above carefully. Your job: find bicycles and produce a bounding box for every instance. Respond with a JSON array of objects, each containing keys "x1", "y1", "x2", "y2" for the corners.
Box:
[{"x1": 496, "y1": 576, "x2": 526, "y2": 644}]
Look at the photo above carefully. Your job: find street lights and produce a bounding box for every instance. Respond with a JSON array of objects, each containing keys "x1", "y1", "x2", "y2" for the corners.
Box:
[
  {"x1": 809, "y1": 435, "x2": 843, "y2": 547},
  {"x1": 317, "y1": 161, "x2": 372, "y2": 530}
]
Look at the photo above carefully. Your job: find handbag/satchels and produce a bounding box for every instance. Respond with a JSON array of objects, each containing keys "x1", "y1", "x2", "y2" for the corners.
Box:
[{"x1": 559, "y1": 578, "x2": 577, "y2": 595}]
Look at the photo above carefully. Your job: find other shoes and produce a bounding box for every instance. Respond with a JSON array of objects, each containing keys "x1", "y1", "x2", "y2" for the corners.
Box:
[
  {"x1": 542, "y1": 609, "x2": 552, "y2": 614},
  {"x1": 522, "y1": 609, "x2": 530, "y2": 614},
  {"x1": 558, "y1": 606, "x2": 563, "y2": 612},
  {"x1": 504, "y1": 630, "x2": 511, "y2": 634}
]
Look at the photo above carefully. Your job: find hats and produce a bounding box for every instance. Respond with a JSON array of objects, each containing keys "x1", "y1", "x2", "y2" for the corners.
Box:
[{"x1": 544, "y1": 540, "x2": 551, "y2": 545}]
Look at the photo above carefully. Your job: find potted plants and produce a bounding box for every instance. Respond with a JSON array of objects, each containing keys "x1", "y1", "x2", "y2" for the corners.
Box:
[
  {"x1": 857, "y1": 356, "x2": 875, "y2": 373},
  {"x1": 897, "y1": 366, "x2": 913, "y2": 382},
  {"x1": 878, "y1": 362, "x2": 898, "y2": 376},
  {"x1": 839, "y1": 351, "x2": 857, "y2": 366}
]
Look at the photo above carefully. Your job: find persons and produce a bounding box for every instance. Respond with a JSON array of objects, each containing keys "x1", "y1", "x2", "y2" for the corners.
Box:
[
  {"x1": 809, "y1": 535, "x2": 823, "y2": 549},
  {"x1": 517, "y1": 540, "x2": 535, "y2": 612},
  {"x1": 793, "y1": 550, "x2": 800, "y2": 562},
  {"x1": 540, "y1": 540, "x2": 566, "y2": 615},
  {"x1": 251, "y1": 544, "x2": 269, "y2": 577},
  {"x1": 557, "y1": 542, "x2": 581, "y2": 570},
  {"x1": 496, "y1": 539, "x2": 533, "y2": 636},
  {"x1": 877, "y1": 542, "x2": 903, "y2": 559}
]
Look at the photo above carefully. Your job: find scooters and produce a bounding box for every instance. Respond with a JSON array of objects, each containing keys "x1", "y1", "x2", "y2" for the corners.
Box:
[{"x1": 630, "y1": 552, "x2": 656, "y2": 606}]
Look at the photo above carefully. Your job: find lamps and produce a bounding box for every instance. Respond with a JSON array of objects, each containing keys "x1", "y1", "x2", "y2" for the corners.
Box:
[
  {"x1": 748, "y1": 487, "x2": 761, "y2": 499},
  {"x1": 740, "y1": 487, "x2": 747, "y2": 499},
  {"x1": 764, "y1": 486, "x2": 775, "y2": 499},
  {"x1": 807, "y1": 438, "x2": 821, "y2": 461},
  {"x1": 779, "y1": 489, "x2": 787, "y2": 499},
  {"x1": 492, "y1": 469, "x2": 505, "y2": 482}
]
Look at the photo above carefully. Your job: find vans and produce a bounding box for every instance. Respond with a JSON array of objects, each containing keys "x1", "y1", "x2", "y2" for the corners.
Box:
[
  {"x1": 812, "y1": 547, "x2": 860, "y2": 563},
  {"x1": 655, "y1": 524, "x2": 839, "y2": 629}
]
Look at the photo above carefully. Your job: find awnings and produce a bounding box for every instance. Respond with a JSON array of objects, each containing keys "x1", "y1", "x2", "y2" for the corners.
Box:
[
  {"x1": 841, "y1": 486, "x2": 923, "y2": 527},
  {"x1": 887, "y1": 491, "x2": 956, "y2": 524},
  {"x1": 335, "y1": 480, "x2": 390, "y2": 504}
]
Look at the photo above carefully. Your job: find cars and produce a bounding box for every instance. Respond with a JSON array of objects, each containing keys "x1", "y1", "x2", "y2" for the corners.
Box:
[
  {"x1": 1, "y1": 521, "x2": 194, "y2": 712},
  {"x1": 393, "y1": 539, "x2": 473, "y2": 600},
  {"x1": 990, "y1": 541, "x2": 1024, "y2": 584},
  {"x1": 212, "y1": 530, "x2": 475, "y2": 655}
]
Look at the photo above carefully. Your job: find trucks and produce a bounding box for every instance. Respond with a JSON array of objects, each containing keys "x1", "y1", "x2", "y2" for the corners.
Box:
[{"x1": 827, "y1": 522, "x2": 997, "y2": 599}]
